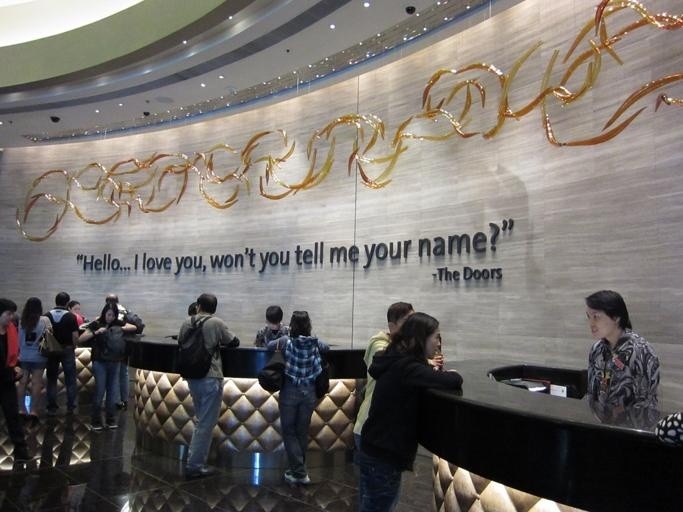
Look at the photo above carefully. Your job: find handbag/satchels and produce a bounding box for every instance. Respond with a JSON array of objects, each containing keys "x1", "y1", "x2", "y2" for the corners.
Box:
[
  {"x1": 315, "y1": 362, "x2": 333, "y2": 398},
  {"x1": 258, "y1": 350, "x2": 287, "y2": 393},
  {"x1": 37, "y1": 330, "x2": 64, "y2": 358}
]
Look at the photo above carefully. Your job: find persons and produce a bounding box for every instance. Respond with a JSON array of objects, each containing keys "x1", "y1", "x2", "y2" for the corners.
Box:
[
  {"x1": 0, "y1": 292, "x2": 145, "y2": 462},
  {"x1": 188, "y1": 302, "x2": 198, "y2": 316},
  {"x1": 177, "y1": 293, "x2": 240, "y2": 481},
  {"x1": 267, "y1": 310, "x2": 329, "y2": 485},
  {"x1": 358, "y1": 312, "x2": 463, "y2": 511},
  {"x1": 580, "y1": 290, "x2": 660, "y2": 410},
  {"x1": 654, "y1": 412, "x2": 683, "y2": 444},
  {"x1": 254, "y1": 305, "x2": 292, "y2": 348},
  {"x1": 353, "y1": 301, "x2": 415, "y2": 455}
]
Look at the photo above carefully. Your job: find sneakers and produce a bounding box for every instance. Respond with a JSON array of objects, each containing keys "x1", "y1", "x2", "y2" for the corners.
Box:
[
  {"x1": 284, "y1": 469, "x2": 301, "y2": 484},
  {"x1": 66, "y1": 408, "x2": 75, "y2": 415},
  {"x1": 105, "y1": 420, "x2": 119, "y2": 430},
  {"x1": 13, "y1": 451, "x2": 38, "y2": 466},
  {"x1": 88, "y1": 419, "x2": 105, "y2": 431},
  {"x1": 299, "y1": 473, "x2": 312, "y2": 485},
  {"x1": 46, "y1": 406, "x2": 57, "y2": 416}
]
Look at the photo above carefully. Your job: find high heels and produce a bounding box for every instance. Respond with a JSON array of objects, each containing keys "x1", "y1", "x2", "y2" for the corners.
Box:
[
  {"x1": 17, "y1": 411, "x2": 27, "y2": 425},
  {"x1": 28, "y1": 412, "x2": 40, "y2": 428}
]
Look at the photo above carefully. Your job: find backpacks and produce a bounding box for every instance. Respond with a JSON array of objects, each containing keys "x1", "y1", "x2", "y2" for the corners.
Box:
[
  {"x1": 173, "y1": 326, "x2": 211, "y2": 380},
  {"x1": 105, "y1": 324, "x2": 129, "y2": 363},
  {"x1": 123, "y1": 311, "x2": 144, "y2": 335}
]
